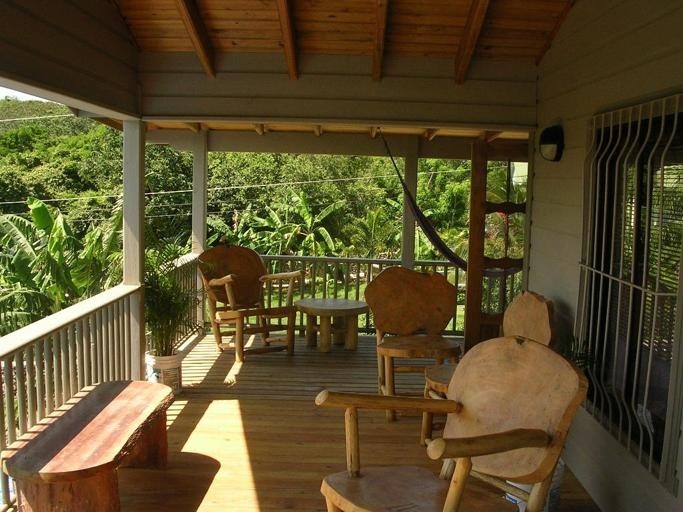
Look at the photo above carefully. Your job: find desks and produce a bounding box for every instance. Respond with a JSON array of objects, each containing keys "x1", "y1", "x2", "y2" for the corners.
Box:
[{"x1": 292, "y1": 296, "x2": 371, "y2": 354}]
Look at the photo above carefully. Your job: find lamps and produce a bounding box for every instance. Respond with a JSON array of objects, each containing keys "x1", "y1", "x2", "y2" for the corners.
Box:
[{"x1": 538, "y1": 115, "x2": 566, "y2": 163}]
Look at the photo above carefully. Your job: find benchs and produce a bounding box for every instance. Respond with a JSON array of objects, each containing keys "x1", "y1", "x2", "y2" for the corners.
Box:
[{"x1": 0, "y1": 378, "x2": 177, "y2": 512}]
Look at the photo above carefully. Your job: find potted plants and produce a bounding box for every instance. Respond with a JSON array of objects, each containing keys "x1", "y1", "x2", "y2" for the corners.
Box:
[{"x1": 140, "y1": 213, "x2": 208, "y2": 396}]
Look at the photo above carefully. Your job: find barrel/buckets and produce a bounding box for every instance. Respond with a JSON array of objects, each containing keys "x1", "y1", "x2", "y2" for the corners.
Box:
[
  {"x1": 144, "y1": 348, "x2": 185, "y2": 396},
  {"x1": 505, "y1": 458, "x2": 565, "y2": 512}
]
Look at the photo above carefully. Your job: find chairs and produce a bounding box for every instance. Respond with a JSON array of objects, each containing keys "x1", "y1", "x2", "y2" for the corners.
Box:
[
  {"x1": 363, "y1": 265, "x2": 464, "y2": 424},
  {"x1": 311, "y1": 332, "x2": 591, "y2": 511},
  {"x1": 196, "y1": 244, "x2": 307, "y2": 365},
  {"x1": 418, "y1": 287, "x2": 556, "y2": 448}
]
